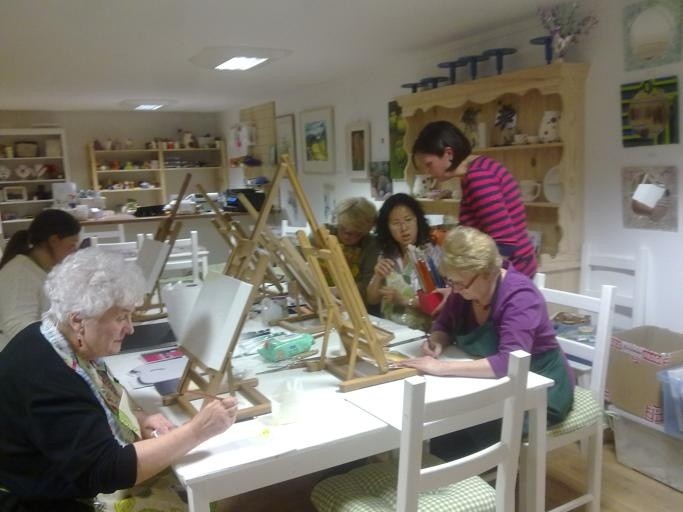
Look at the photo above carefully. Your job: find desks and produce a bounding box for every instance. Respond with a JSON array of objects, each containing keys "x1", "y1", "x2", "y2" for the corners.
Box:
[{"x1": 101, "y1": 284, "x2": 554, "y2": 512}]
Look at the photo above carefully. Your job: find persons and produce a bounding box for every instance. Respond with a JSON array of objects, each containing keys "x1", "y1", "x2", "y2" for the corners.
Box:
[
  {"x1": 365, "y1": 193, "x2": 441, "y2": 333},
  {"x1": 0, "y1": 210, "x2": 82, "y2": 354},
  {"x1": 0, "y1": 246, "x2": 239, "y2": 512},
  {"x1": 413, "y1": 121, "x2": 537, "y2": 319},
  {"x1": 298, "y1": 197, "x2": 384, "y2": 318},
  {"x1": 395, "y1": 225, "x2": 574, "y2": 490}
]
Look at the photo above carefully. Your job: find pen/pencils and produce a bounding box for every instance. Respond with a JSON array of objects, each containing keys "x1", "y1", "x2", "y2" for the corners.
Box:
[
  {"x1": 414, "y1": 254, "x2": 442, "y2": 294},
  {"x1": 184, "y1": 388, "x2": 244, "y2": 406},
  {"x1": 425, "y1": 334, "x2": 439, "y2": 360}
]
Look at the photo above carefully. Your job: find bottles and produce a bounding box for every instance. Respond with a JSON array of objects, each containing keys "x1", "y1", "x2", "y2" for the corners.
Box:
[
  {"x1": 538, "y1": 111, "x2": 560, "y2": 144},
  {"x1": 163, "y1": 142, "x2": 180, "y2": 149},
  {"x1": 124, "y1": 180, "x2": 135, "y2": 189}
]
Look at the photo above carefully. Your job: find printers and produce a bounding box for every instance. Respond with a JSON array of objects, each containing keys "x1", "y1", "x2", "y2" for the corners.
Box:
[{"x1": 223, "y1": 188, "x2": 266, "y2": 212}]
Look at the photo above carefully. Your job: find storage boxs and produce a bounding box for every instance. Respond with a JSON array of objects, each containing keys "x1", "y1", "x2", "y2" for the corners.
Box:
[
  {"x1": 659, "y1": 366, "x2": 683, "y2": 436},
  {"x1": 602, "y1": 325, "x2": 683, "y2": 424},
  {"x1": 609, "y1": 404, "x2": 683, "y2": 494}
]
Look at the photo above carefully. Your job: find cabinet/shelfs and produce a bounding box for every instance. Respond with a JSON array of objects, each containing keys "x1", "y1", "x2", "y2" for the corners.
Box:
[
  {"x1": 89, "y1": 139, "x2": 230, "y2": 206},
  {"x1": 399, "y1": 60, "x2": 588, "y2": 318},
  {"x1": 0, "y1": 127, "x2": 70, "y2": 240}
]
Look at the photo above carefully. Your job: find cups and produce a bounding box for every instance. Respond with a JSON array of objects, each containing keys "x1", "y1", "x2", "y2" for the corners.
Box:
[
  {"x1": 513, "y1": 135, "x2": 528, "y2": 145},
  {"x1": 518, "y1": 180, "x2": 541, "y2": 203},
  {"x1": 528, "y1": 135, "x2": 538, "y2": 143},
  {"x1": 272, "y1": 295, "x2": 289, "y2": 319},
  {"x1": 412, "y1": 174, "x2": 437, "y2": 198}
]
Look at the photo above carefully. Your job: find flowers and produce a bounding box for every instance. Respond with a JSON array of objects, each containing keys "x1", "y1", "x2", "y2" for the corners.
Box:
[{"x1": 539, "y1": 3, "x2": 599, "y2": 61}]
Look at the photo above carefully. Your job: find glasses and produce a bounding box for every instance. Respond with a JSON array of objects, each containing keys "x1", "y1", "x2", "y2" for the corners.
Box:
[{"x1": 442, "y1": 274, "x2": 480, "y2": 289}]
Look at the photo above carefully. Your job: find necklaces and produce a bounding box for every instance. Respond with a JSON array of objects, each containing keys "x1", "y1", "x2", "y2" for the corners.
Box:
[{"x1": 474, "y1": 299, "x2": 494, "y2": 312}]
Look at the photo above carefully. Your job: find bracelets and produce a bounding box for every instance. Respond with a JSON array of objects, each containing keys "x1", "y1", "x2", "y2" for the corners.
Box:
[
  {"x1": 131, "y1": 406, "x2": 144, "y2": 412},
  {"x1": 408, "y1": 297, "x2": 414, "y2": 309}
]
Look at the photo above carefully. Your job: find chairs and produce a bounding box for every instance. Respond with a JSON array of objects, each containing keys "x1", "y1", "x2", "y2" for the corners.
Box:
[
  {"x1": 310, "y1": 350, "x2": 532, "y2": 512},
  {"x1": 282, "y1": 219, "x2": 312, "y2": 238},
  {"x1": 517, "y1": 273, "x2": 617, "y2": 512},
  {"x1": 554, "y1": 243, "x2": 646, "y2": 392},
  {"x1": 78, "y1": 223, "x2": 209, "y2": 284}
]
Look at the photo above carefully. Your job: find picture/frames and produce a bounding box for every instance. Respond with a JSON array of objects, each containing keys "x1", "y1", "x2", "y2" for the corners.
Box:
[
  {"x1": 300, "y1": 105, "x2": 336, "y2": 174},
  {"x1": 275, "y1": 113, "x2": 296, "y2": 165},
  {"x1": 347, "y1": 128, "x2": 370, "y2": 179}
]
